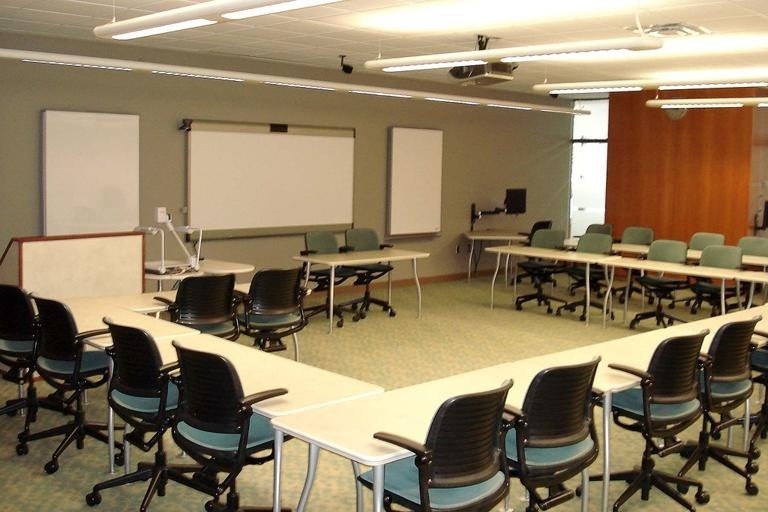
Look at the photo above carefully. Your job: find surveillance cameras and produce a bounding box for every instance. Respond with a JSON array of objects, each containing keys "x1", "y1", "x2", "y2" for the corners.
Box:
[{"x1": 342, "y1": 65, "x2": 354, "y2": 74}]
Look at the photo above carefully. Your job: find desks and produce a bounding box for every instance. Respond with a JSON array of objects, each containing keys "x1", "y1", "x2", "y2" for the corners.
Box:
[
  {"x1": 293, "y1": 248, "x2": 430, "y2": 333},
  {"x1": 270, "y1": 302, "x2": 766, "y2": 512},
  {"x1": 143, "y1": 258, "x2": 255, "y2": 290},
  {"x1": 100, "y1": 283, "x2": 252, "y2": 318},
  {"x1": 26, "y1": 292, "x2": 385, "y2": 512},
  {"x1": 484, "y1": 245, "x2": 765, "y2": 328},
  {"x1": 465, "y1": 228, "x2": 528, "y2": 280}
]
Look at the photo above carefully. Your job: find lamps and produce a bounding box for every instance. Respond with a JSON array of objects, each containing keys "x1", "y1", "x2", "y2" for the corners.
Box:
[
  {"x1": 533, "y1": 71, "x2": 767, "y2": 94},
  {"x1": 90, "y1": 0, "x2": 348, "y2": 43},
  {"x1": 642, "y1": 96, "x2": 768, "y2": 112},
  {"x1": 362, "y1": 32, "x2": 661, "y2": 73}
]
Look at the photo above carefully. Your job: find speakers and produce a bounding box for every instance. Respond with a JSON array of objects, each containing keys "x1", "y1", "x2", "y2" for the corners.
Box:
[{"x1": 506, "y1": 188, "x2": 526, "y2": 213}]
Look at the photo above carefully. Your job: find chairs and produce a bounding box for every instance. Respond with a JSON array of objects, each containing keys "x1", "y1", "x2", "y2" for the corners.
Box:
[
  {"x1": 236, "y1": 262, "x2": 308, "y2": 349},
  {"x1": 337, "y1": 227, "x2": 397, "y2": 320},
  {"x1": 511, "y1": 220, "x2": 766, "y2": 330},
  {"x1": 356, "y1": 378, "x2": 513, "y2": 512},
  {"x1": 501, "y1": 354, "x2": 606, "y2": 512},
  {"x1": 590, "y1": 326, "x2": 711, "y2": 512},
  {"x1": 80, "y1": 313, "x2": 243, "y2": 511},
  {"x1": 15, "y1": 291, "x2": 139, "y2": 474},
  {"x1": 657, "y1": 314, "x2": 764, "y2": 495},
  {"x1": 0, "y1": 285, "x2": 79, "y2": 418},
  {"x1": 170, "y1": 337, "x2": 298, "y2": 512},
  {"x1": 169, "y1": 274, "x2": 240, "y2": 341},
  {"x1": 300, "y1": 232, "x2": 367, "y2": 327}
]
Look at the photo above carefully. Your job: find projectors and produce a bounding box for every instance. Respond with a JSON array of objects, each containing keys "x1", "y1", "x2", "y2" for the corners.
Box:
[{"x1": 449, "y1": 62, "x2": 514, "y2": 87}]
